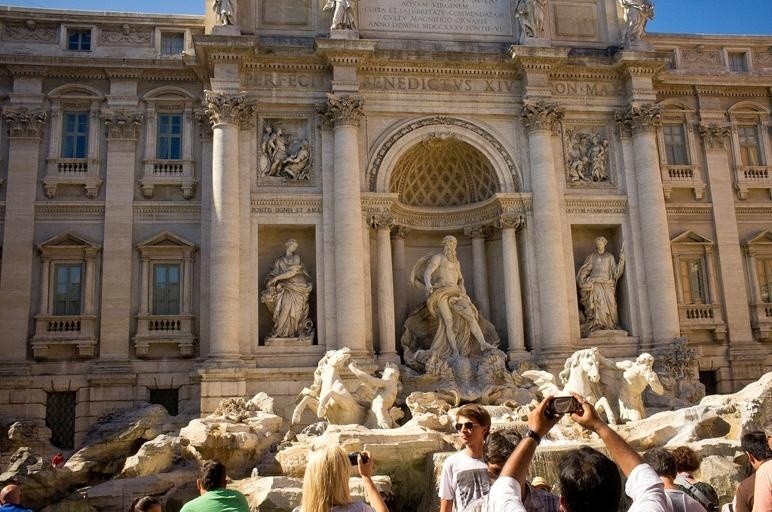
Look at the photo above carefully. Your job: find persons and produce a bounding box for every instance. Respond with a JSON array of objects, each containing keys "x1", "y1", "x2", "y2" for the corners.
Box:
[
  {"x1": 260, "y1": 235, "x2": 321, "y2": 344},
  {"x1": 566, "y1": 129, "x2": 609, "y2": 187},
  {"x1": 513, "y1": 2, "x2": 550, "y2": 40},
  {"x1": 348, "y1": 359, "x2": 400, "y2": 430},
  {"x1": 409, "y1": 229, "x2": 504, "y2": 360},
  {"x1": 573, "y1": 228, "x2": 626, "y2": 333},
  {"x1": 619, "y1": 1, "x2": 659, "y2": 38},
  {"x1": 292, "y1": 444, "x2": 390, "y2": 512},
  {"x1": 213, "y1": 1, "x2": 239, "y2": 26},
  {"x1": 321, "y1": 0, "x2": 359, "y2": 32},
  {"x1": 52, "y1": 453, "x2": 64, "y2": 469},
  {"x1": 437, "y1": 393, "x2": 668, "y2": 512},
  {"x1": 593, "y1": 346, "x2": 663, "y2": 423},
  {"x1": 263, "y1": 124, "x2": 309, "y2": 181},
  {"x1": 180, "y1": 462, "x2": 251, "y2": 512},
  {"x1": 642, "y1": 431, "x2": 772, "y2": 512},
  {"x1": 0, "y1": 485, "x2": 32, "y2": 512},
  {"x1": 131, "y1": 496, "x2": 161, "y2": 512}
]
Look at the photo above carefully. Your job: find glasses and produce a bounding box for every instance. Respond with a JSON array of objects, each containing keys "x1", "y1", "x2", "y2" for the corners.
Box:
[{"x1": 455, "y1": 422, "x2": 481, "y2": 429}]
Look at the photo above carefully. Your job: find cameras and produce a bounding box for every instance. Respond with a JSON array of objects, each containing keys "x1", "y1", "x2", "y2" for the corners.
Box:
[
  {"x1": 348, "y1": 452, "x2": 368, "y2": 466},
  {"x1": 549, "y1": 396, "x2": 580, "y2": 416}
]
